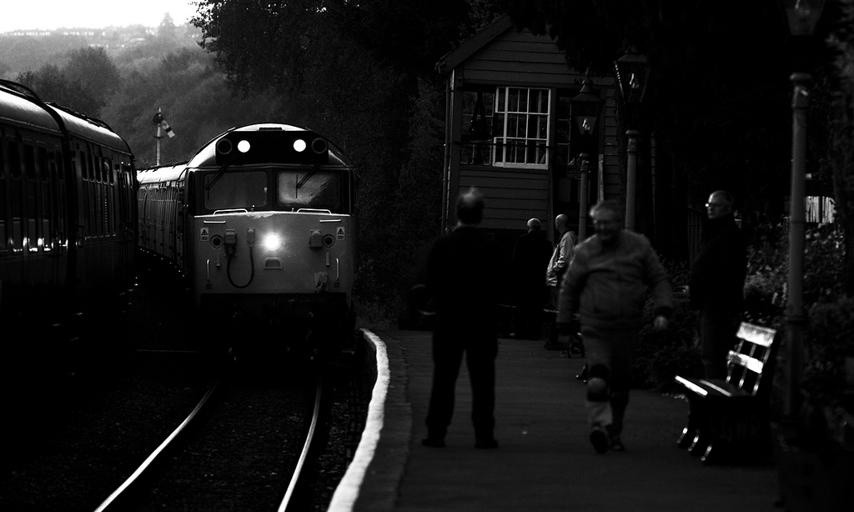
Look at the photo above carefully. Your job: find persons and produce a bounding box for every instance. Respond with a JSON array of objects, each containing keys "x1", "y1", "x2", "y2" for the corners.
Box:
[
  {"x1": 689, "y1": 189, "x2": 746, "y2": 379},
  {"x1": 508, "y1": 217, "x2": 550, "y2": 340},
  {"x1": 544, "y1": 212, "x2": 577, "y2": 349},
  {"x1": 420, "y1": 188, "x2": 505, "y2": 449},
  {"x1": 555, "y1": 199, "x2": 674, "y2": 455}
]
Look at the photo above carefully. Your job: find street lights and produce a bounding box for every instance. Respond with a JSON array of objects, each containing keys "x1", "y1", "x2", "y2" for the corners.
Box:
[
  {"x1": 785, "y1": 0, "x2": 826, "y2": 414},
  {"x1": 568, "y1": 78, "x2": 606, "y2": 243},
  {"x1": 614, "y1": 45, "x2": 652, "y2": 232}
]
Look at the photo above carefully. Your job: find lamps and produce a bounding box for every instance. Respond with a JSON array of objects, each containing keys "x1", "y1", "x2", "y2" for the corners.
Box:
[{"x1": 569, "y1": 65, "x2": 601, "y2": 106}]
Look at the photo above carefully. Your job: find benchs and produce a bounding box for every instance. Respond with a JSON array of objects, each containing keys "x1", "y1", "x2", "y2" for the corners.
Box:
[{"x1": 674, "y1": 319, "x2": 777, "y2": 469}]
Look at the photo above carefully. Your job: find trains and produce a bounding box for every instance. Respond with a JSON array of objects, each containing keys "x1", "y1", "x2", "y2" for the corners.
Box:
[
  {"x1": 134, "y1": 121, "x2": 359, "y2": 350},
  {"x1": 0, "y1": 77, "x2": 137, "y2": 288}
]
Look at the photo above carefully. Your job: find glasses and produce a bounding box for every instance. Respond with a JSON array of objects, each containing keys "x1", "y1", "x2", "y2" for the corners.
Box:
[{"x1": 705, "y1": 202, "x2": 721, "y2": 209}]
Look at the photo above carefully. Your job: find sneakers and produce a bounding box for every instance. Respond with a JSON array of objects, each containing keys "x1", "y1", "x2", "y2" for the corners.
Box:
[
  {"x1": 421, "y1": 436, "x2": 445, "y2": 448},
  {"x1": 474, "y1": 438, "x2": 499, "y2": 449},
  {"x1": 588, "y1": 423, "x2": 625, "y2": 455}
]
